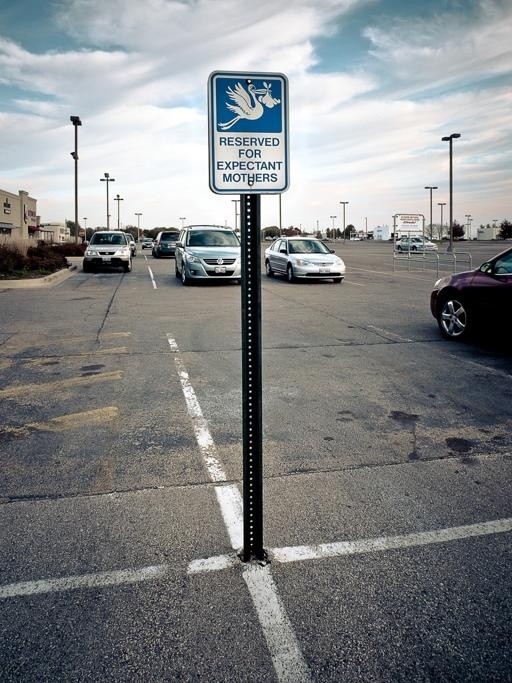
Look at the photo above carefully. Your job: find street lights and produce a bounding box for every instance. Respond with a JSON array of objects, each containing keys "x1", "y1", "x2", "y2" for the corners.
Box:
[
  {"x1": 177, "y1": 216, "x2": 186, "y2": 226},
  {"x1": 424, "y1": 184, "x2": 438, "y2": 242},
  {"x1": 83, "y1": 217, "x2": 89, "y2": 242},
  {"x1": 340, "y1": 201, "x2": 348, "y2": 239},
  {"x1": 68, "y1": 114, "x2": 82, "y2": 245},
  {"x1": 492, "y1": 219, "x2": 498, "y2": 239},
  {"x1": 135, "y1": 212, "x2": 143, "y2": 243},
  {"x1": 112, "y1": 191, "x2": 124, "y2": 230},
  {"x1": 100, "y1": 172, "x2": 115, "y2": 231},
  {"x1": 327, "y1": 214, "x2": 337, "y2": 238},
  {"x1": 436, "y1": 202, "x2": 446, "y2": 244},
  {"x1": 463, "y1": 214, "x2": 473, "y2": 240},
  {"x1": 440, "y1": 131, "x2": 461, "y2": 250}
]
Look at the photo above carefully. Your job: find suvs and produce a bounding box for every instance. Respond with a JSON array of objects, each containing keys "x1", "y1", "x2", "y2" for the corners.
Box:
[
  {"x1": 174, "y1": 223, "x2": 241, "y2": 286},
  {"x1": 151, "y1": 230, "x2": 181, "y2": 258},
  {"x1": 81, "y1": 228, "x2": 133, "y2": 273}
]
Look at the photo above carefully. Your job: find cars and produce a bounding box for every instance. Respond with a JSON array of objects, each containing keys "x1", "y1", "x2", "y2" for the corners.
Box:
[
  {"x1": 265, "y1": 235, "x2": 273, "y2": 240},
  {"x1": 350, "y1": 235, "x2": 362, "y2": 241},
  {"x1": 124, "y1": 232, "x2": 137, "y2": 256},
  {"x1": 430, "y1": 244, "x2": 512, "y2": 345},
  {"x1": 395, "y1": 235, "x2": 438, "y2": 254},
  {"x1": 141, "y1": 236, "x2": 154, "y2": 249},
  {"x1": 263, "y1": 235, "x2": 346, "y2": 285},
  {"x1": 271, "y1": 235, "x2": 279, "y2": 240}
]
